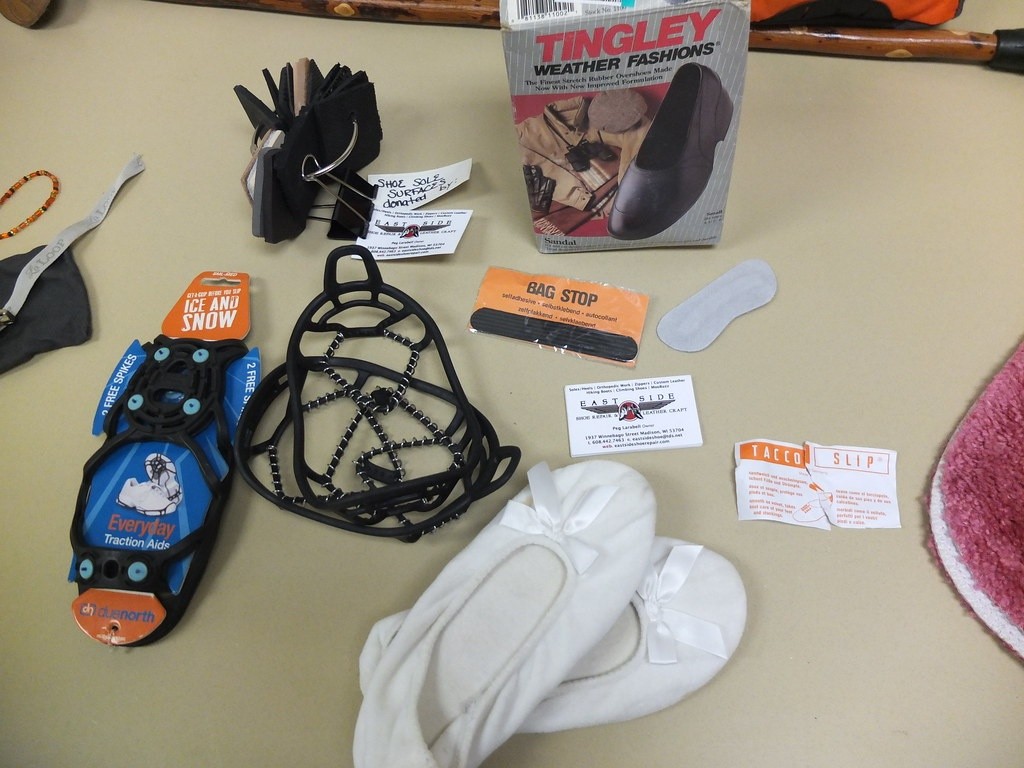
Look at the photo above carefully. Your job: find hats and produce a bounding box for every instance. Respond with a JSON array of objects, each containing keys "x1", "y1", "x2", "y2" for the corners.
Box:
[{"x1": 588, "y1": 88, "x2": 648, "y2": 135}]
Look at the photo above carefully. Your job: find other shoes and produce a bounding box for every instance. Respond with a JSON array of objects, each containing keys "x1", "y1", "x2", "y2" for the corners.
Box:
[
  {"x1": 358, "y1": 536, "x2": 746, "y2": 738},
  {"x1": 353, "y1": 459, "x2": 658, "y2": 768}
]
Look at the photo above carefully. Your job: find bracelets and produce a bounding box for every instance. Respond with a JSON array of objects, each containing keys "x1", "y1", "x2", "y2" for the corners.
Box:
[{"x1": 0, "y1": 170, "x2": 59, "y2": 239}]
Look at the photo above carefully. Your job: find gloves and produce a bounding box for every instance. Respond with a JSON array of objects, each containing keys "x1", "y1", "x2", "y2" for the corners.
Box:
[{"x1": 522, "y1": 165, "x2": 556, "y2": 221}]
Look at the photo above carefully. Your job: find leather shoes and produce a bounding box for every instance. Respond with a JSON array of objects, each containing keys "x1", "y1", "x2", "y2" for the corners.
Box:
[{"x1": 606, "y1": 62, "x2": 734, "y2": 240}]
